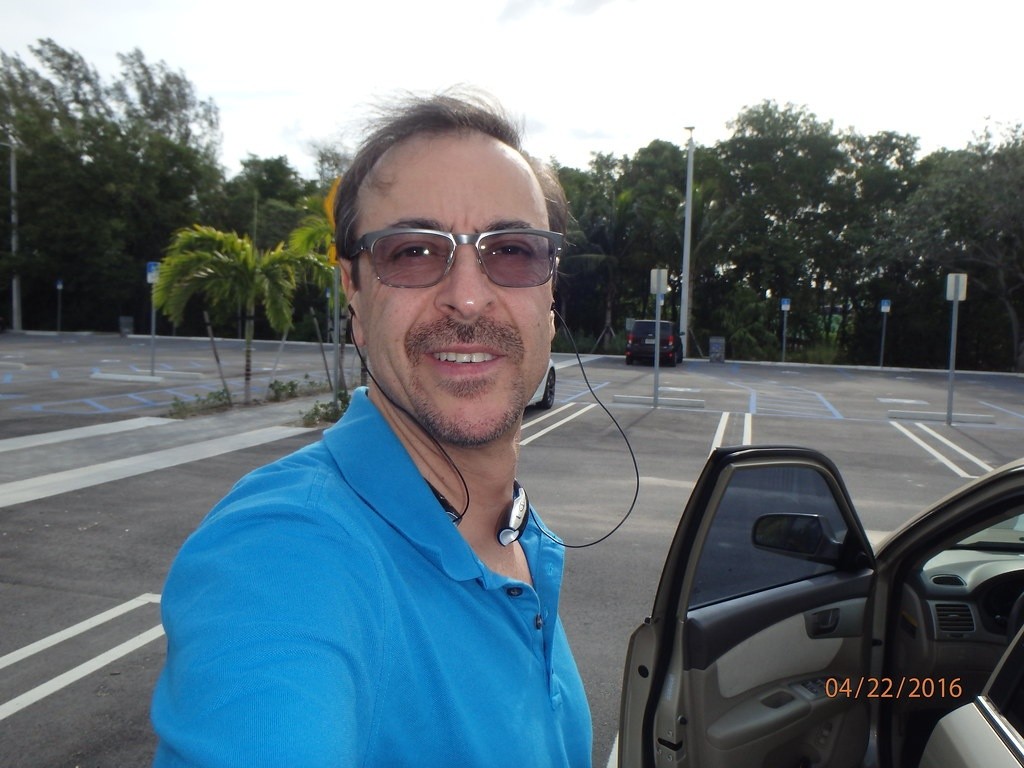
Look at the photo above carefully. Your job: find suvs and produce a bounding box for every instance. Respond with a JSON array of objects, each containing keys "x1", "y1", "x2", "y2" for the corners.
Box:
[{"x1": 626, "y1": 320, "x2": 684, "y2": 367}]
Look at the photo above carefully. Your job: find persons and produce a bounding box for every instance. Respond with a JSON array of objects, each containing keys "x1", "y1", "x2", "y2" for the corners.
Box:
[{"x1": 149, "y1": 94, "x2": 593, "y2": 768}]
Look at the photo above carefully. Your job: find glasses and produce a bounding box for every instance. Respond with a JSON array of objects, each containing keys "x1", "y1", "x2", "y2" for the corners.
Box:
[{"x1": 349, "y1": 226, "x2": 565, "y2": 290}]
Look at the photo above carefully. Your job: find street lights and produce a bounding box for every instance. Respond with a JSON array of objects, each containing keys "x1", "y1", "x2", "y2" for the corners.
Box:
[{"x1": 680, "y1": 125, "x2": 695, "y2": 358}]
